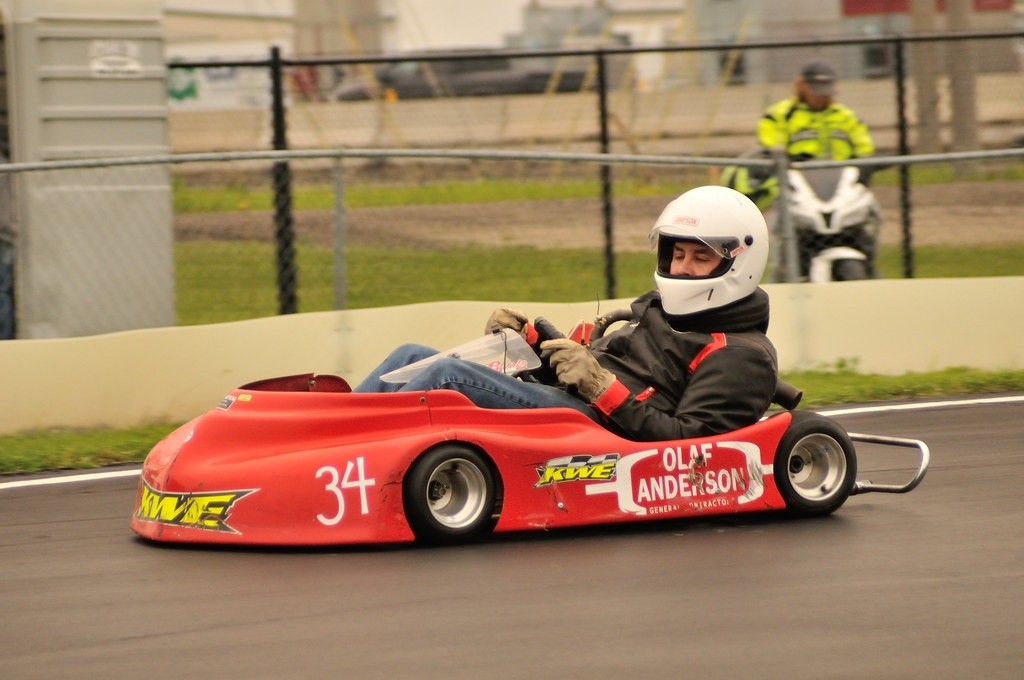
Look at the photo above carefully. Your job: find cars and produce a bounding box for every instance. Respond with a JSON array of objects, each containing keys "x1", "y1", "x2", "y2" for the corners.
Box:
[{"x1": 332, "y1": 44, "x2": 587, "y2": 101}]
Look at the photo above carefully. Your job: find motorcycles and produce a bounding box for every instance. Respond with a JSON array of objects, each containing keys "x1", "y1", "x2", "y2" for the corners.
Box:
[{"x1": 766, "y1": 152, "x2": 896, "y2": 282}]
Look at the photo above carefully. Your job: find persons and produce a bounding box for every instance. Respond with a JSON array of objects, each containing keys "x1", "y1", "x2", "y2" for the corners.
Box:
[
  {"x1": 352, "y1": 186, "x2": 777, "y2": 442},
  {"x1": 758, "y1": 62, "x2": 875, "y2": 205}
]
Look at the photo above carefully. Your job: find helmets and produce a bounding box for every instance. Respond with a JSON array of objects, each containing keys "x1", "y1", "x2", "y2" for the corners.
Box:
[{"x1": 648, "y1": 187, "x2": 770, "y2": 319}]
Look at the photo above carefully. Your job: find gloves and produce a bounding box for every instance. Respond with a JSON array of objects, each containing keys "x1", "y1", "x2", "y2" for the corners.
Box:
[
  {"x1": 540, "y1": 338, "x2": 616, "y2": 405},
  {"x1": 485, "y1": 308, "x2": 529, "y2": 344}
]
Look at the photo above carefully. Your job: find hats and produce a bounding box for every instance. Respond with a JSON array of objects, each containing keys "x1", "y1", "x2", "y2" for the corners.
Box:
[{"x1": 800, "y1": 61, "x2": 840, "y2": 99}]
